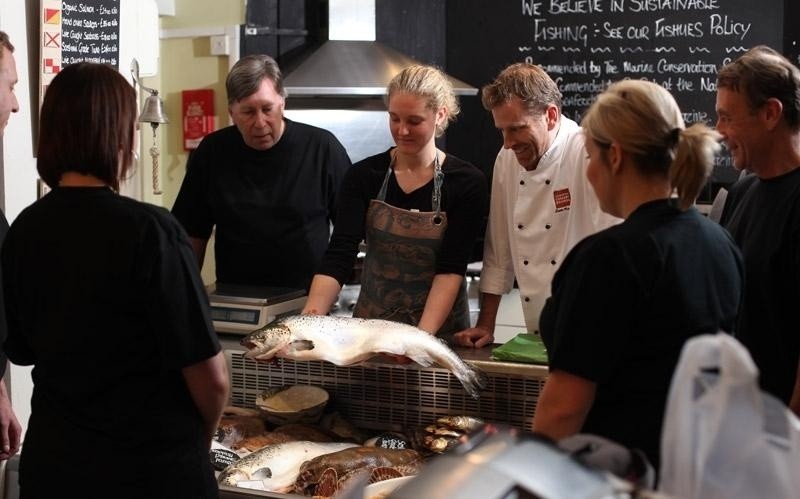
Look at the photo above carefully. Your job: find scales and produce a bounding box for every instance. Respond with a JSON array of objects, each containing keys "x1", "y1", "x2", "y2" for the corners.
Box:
[{"x1": 203, "y1": 279, "x2": 308, "y2": 336}]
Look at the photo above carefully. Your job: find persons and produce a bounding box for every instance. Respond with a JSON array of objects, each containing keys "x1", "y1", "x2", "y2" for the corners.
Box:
[
  {"x1": 172, "y1": 53, "x2": 357, "y2": 288},
  {"x1": 1, "y1": 32, "x2": 24, "y2": 465},
  {"x1": 302, "y1": 61, "x2": 490, "y2": 343},
  {"x1": 450, "y1": 59, "x2": 622, "y2": 351},
  {"x1": 1, "y1": 63, "x2": 228, "y2": 495},
  {"x1": 532, "y1": 79, "x2": 753, "y2": 494},
  {"x1": 713, "y1": 46, "x2": 800, "y2": 415}
]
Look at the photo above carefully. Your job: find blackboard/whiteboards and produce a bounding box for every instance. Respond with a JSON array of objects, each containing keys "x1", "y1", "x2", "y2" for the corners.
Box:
[
  {"x1": 444, "y1": 0, "x2": 800, "y2": 184},
  {"x1": 61, "y1": 1, "x2": 120, "y2": 73}
]
